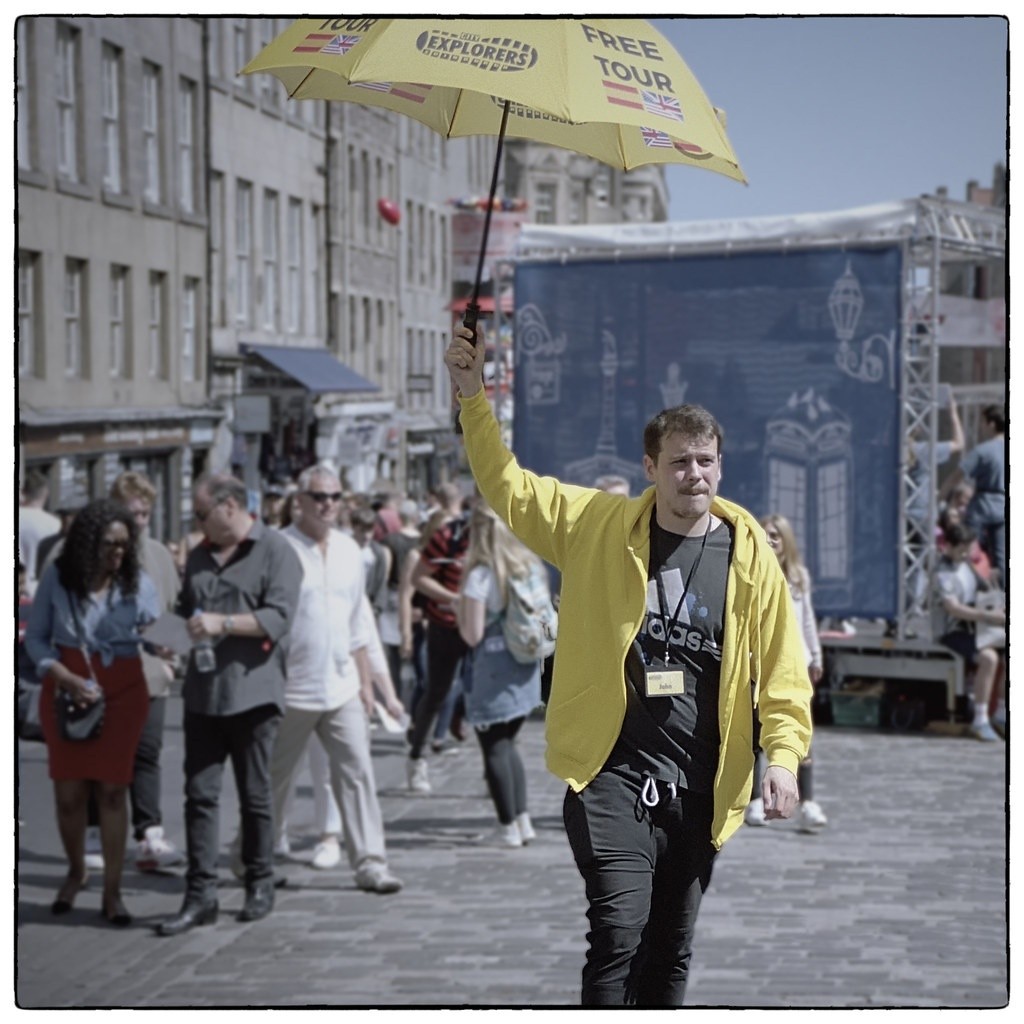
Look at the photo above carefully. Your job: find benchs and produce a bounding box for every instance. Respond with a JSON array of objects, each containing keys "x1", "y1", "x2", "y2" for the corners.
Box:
[{"x1": 817, "y1": 630, "x2": 967, "y2": 733}]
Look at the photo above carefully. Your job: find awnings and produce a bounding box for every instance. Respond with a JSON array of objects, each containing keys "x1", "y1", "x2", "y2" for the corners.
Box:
[{"x1": 239, "y1": 339, "x2": 381, "y2": 392}]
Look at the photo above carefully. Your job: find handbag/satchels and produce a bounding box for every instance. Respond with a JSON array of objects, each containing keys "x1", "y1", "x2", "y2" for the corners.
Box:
[
  {"x1": 54, "y1": 685, "x2": 108, "y2": 743},
  {"x1": 964, "y1": 562, "x2": 1006, "y2": 650}
]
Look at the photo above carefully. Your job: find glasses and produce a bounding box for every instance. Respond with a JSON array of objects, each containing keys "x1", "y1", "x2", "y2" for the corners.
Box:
[
  {"x1": 195, "y1": 496, "x2": 227, "y2": 522},
  {"x1": 302, "y1": 490, "x2": 343, "y2": 503}
]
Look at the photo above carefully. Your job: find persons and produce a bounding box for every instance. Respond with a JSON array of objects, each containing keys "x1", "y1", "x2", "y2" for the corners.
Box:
[
  {"x1": 908, "y1": 400, "x2": 1005, "y2": 740},
  {"x1": 744, "y1": 514, "x2": 827, "y2": 833},
  {"x1": 443, "y1": 322, "x2": 814, "y2": 1004},
  {"x1": 18, "y1": 465, "x2": 628, "y2": 940}
]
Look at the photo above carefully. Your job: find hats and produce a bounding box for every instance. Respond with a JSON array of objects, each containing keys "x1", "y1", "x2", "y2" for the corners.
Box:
[
  {"x1": 261, "y1": 484, "x2": 283, "y2": 496},
  {"x1": 938, "y1": 524, "x2": 976, "y2": 544}
]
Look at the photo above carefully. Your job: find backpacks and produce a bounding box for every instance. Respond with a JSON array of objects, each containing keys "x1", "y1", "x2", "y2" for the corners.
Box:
[{"x1": 502, "y1": 567, "x2": 559, "y2": 664}]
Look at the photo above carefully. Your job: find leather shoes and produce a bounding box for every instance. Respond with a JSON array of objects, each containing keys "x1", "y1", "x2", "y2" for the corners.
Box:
[
  {"x1": 236, "y1": 882, "x2": 275, "y2": 921},
  {"x1": 153, "y1": 896, "x2": 218, "y2": 936}
]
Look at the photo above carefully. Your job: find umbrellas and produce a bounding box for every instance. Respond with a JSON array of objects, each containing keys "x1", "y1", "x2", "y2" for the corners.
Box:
[{"x1": 236, "y1": 19, "x2": 750, "y2": 369}]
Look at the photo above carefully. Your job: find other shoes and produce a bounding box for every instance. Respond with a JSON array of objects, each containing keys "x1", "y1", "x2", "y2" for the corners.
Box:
[
  {"x1": 310, "y1": 841, "x2": 342, "y2": 869},
  {"x1": 84, "y1": 826, "x2": 105, "y2": 869},
  {"x1": 233, "y1": 860, "x2": 287, "y2": 887},
  {"x1": 746, "y1": 798, "x2": 770, "y2": 826},
  {"x1": 516, "y1": 811, "x2": 535, "y2": 842},
  {"x1": 969, "y1": 720, "x2": 998, "y2": 741},
  {"x1": 270, "y1": 830, "x2": 290, "y2": 859},
  {"x1": 135, "y1": 826, "x2": 183, "y2": 869},
  {"x1": 355, "y1": 863, "x2": 402, "y2": 895},
  {"x1": 406, "y1": 757, "x2": 429, "y2": 791},
  {"x1": 101, "y1": 893, "x2": 131, "y2": 926},
  {"x1": 796, "y1": 800, "x2": 827, "y2": 831},
  {"x1": 433, "y1": 737, "x2": 458, "y2": 757},
  {"x1": 468, "y1": 821, "x2": 522, "y2": 847},
  {"x1": 50, "y1": 872, "x2": 89, "y2": 917}
]
